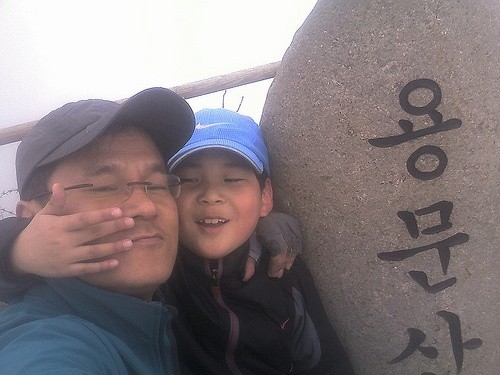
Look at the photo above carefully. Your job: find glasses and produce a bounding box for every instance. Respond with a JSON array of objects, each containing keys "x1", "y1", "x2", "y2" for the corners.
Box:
[{"x1": 24, "y1": 172, "x2": 182, "y2": 202}]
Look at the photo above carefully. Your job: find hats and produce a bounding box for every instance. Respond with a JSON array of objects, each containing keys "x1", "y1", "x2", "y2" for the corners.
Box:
[
  {"x1": 167, "y1": 108, "x2": 273, "y2": 176},
  {"x1": 15, "y1": 86, "x2": 198, "y2": 199}
]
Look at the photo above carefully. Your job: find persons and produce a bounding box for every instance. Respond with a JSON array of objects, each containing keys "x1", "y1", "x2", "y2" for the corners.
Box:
[
  {"x1": 0, "y1": 87, "x2": 303, "y2": 375},
  {"x1": 1, "y1": 109, "x2": 354, "y2": 375}
]
